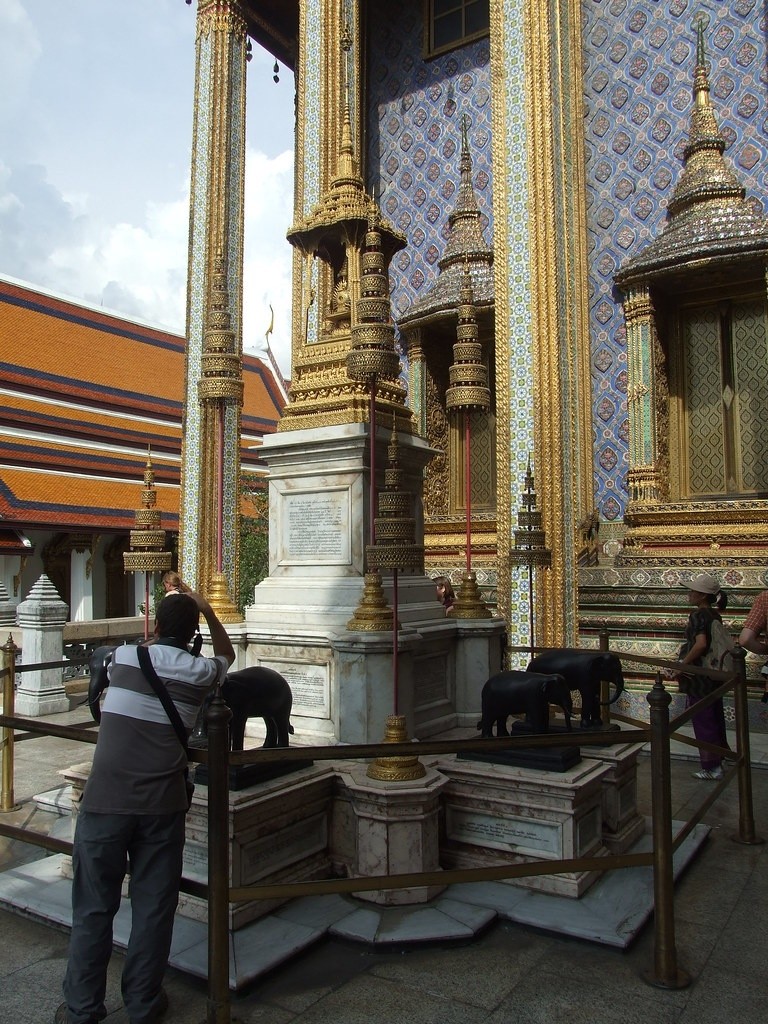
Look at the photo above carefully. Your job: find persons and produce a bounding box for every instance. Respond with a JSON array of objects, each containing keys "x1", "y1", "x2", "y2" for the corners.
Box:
[
  {"x1": 432, "y1": 577, "x2": 457, "y2": 615},
  {"x1": 664, "y1": 575, "x2": 736, "y2": 780},
  {"x1": 739, "y1": 590, "x2": 768, "y2": 655},
  {"x1": 760, "y1": 661, "x2": 768, "y2": 704},
  {"x1": 53, "y1": 591, "x2": 236, "y2": 1024},
  {"x1": 164, "y1": 571, "x2": 192, "y2": 597}
]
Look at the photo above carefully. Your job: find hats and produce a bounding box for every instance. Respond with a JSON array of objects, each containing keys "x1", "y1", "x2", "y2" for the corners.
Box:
[{"x1": 678, "y1": 575, "x2": 721, "y2": 595}]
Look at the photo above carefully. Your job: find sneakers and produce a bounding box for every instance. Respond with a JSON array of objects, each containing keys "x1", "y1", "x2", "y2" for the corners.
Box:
[
  {"x1": 721, "y1": 760, "x2": 727, "y2": 771},
  {"x1": 691, "y1": 766, "x2": 724, "y2": 779},
  {"x1": 55, "y1": 1002, "x2": 98, "y2": 1024},
  {"x1": 129, "y1": 986, "x2": 168, "y2": 1024}
]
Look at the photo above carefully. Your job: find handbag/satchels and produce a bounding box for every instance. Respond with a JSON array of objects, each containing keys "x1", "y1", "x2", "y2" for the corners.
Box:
[{"x1": 182, "y1": 766, "x2": 195, "y2": 813}]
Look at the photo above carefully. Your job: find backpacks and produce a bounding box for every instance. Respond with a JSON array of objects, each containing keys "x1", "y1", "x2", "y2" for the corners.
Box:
[{"x1": 704, "y1": 607, "x2": 735, "y2": 678}]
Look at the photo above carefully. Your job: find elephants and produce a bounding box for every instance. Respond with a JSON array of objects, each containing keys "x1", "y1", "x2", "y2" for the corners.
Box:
[
  {"x1": 220, "y1": 666, "x2": 294, "y2": 750},
  {"x1": 476, "y1": 670, "x2": 578, "y2": 737},
  {"x1": 527, "y1": 649, "x2": 630, "y2": 730}
]
